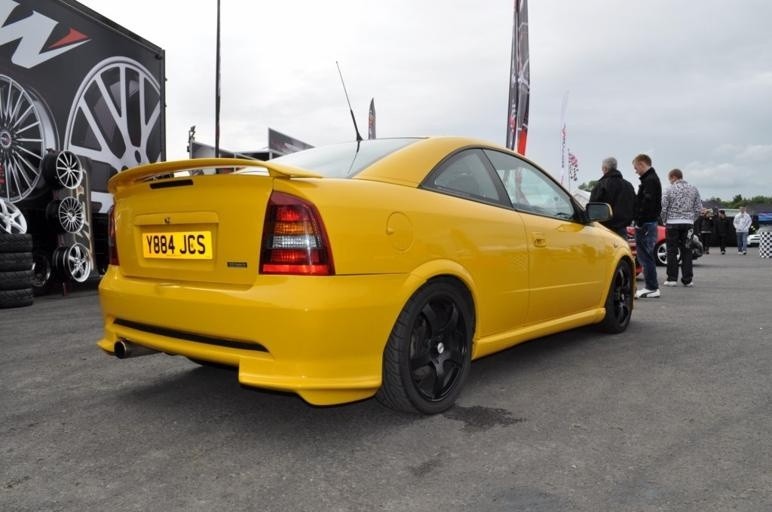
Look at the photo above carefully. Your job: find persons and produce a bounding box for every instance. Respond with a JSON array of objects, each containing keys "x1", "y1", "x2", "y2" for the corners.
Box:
[
  {"x1": 699, "y1": 218, "x2": 712, "y2": 255},
  {"x1": 589, "y1": 158, "x2": 635, "y2": 239},
  {"x1": 661, "y1": 168, "x2": 702, "y2": 288},
  {"x1": 716, "y1": 210, "x2": 728, "y2": 254},
  {"x1": 732, "y1": 205, "x2": 752, "y2": 255},
  {"x1": 631, "y1": 154, "x2": 661, "y2": 297}
]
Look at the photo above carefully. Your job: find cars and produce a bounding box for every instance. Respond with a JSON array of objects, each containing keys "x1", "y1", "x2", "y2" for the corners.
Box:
[{"x1": 746, "y1": 224, "x2": 772, "y2": 247}]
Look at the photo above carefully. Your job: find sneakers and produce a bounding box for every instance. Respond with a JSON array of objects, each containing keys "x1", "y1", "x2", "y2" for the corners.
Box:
[
  {"x1": 703, "y1": 251, "x2": 710, "y2": 255},
  {"x1": 684, "y1": 282, "x2": 694, "y2": 287},
  {"x1": 721, "y1": 249, "x2": 725, "y2": 255},
  {"x1": 635, "y1": 289, "x2": 660, "y2": 297},
  {"x1": 738, "y1": 250, "x2": 747, "y2": 255},
  {"x1": 663, "y1": 281, "x2": 677, "y2": 287}
]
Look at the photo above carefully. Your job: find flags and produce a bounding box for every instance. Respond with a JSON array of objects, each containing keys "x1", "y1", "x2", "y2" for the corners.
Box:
[{"x1": 568, "y1": 153, "x2": 577, "y2": 168}]
